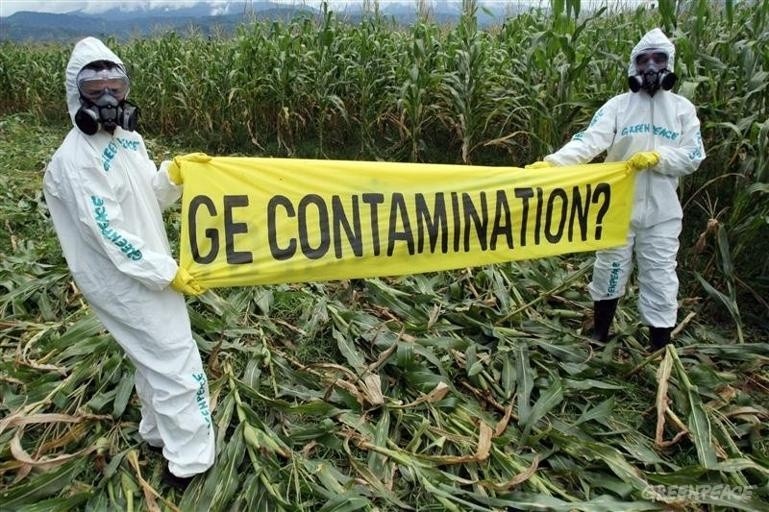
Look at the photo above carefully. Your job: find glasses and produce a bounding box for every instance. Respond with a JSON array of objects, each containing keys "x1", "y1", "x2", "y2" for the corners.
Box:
[
  {"x1": 80, "y1": 77, "x2": 129, "y2": 101},
  {"x1": 634, "y1": 53, "x2": 670, "y2": 71}
]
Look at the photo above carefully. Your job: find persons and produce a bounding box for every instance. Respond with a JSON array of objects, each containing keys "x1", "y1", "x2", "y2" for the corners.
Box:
[
  {"x1": 42, "y1": 36, "x2": 219, "y2": 488},
  {"x1": 524, "y1": 26, "x2": 707, "y2": 355}
]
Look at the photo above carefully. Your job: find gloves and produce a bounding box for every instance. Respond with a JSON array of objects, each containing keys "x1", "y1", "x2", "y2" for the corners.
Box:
[
  {"x1": 169, "y1": 267, "x2": 209, "y2": 296},
  {"x1": 164, "y1": 151, "x2": 213, "y2": 187},
  {"x1": 626, "y1": 151, "x2": 660, "y2": 172},
  {"x1": 525, "y1": 160, "x2": 556, "y2": 172}
]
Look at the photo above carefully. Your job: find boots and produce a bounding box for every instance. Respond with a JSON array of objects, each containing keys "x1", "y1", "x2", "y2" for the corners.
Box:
[
  {"x1": 590, "y1": 298, "x2": 619, "y2": 348},
  {"x1": 646, "y1": 325, "x2": 671, "y2": 357}
]
